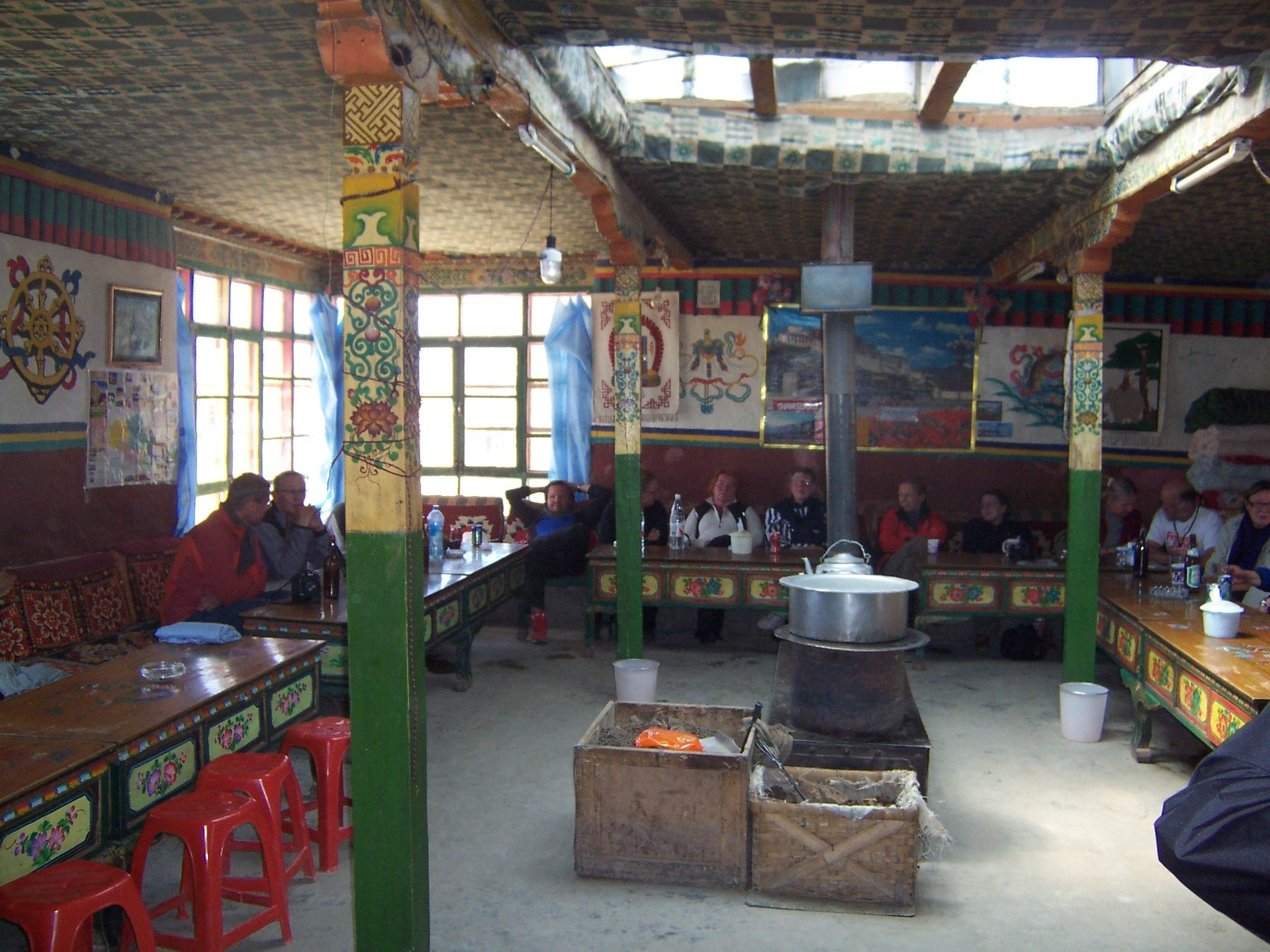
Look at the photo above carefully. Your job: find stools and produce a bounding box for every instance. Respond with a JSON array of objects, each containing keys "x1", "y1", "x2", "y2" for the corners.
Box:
[
  {"x1": 277, "y1": 716, "x2": 354, "y2": 874},
  {"x1": 0, "y1": 861, "x2": 156, "y2": 952},
  {"x1": 118, "y1": 788, "x2": 294, "y2": 952},
  {"x1": 175, "y1": 751, "x2": 318, "y2": 920}
]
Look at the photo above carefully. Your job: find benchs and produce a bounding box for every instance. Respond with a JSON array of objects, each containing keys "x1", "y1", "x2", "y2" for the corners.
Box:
[
  {"x1": 0, "y1": 536, "x2": 181, "y2": 698},
  {"x1": 913, "y1": 553, "x2": 1069, "y2": 659},
  {"x1": 420, "y1": 495, "x2": 503, "y2": 543}
]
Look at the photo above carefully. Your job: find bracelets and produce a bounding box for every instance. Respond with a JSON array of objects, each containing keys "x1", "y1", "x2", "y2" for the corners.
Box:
[
  {"x1": 577, "y1": 484, "x2": 584, "y2": 492},
  {"x1": 1260, "y1": 599, "x2": 1270, "y2": 612}
]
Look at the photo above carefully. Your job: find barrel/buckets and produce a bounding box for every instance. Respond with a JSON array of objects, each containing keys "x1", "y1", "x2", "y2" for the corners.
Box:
[{"x1": 1200, "y1": 600, "x2": 1244, "y2": 639}]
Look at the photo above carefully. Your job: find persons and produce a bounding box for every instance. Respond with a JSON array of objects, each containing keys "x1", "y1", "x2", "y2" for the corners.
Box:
[
  {"x1": 757, "y1": 466, "x2": 828, "y2": 629},
  {"x1": 255, "y1": 471, "x2": 330, "y2": 596},
  {"x1": 307, "y1": 503, "x2": 347, "y2": 598},
  {"x1": 506, "y1": 480, "x2": 615, "y2": 643},
  {"x1": 873, "y1": 475, "x2": 948, "y2": 609},
  {"x1": 1146, "y1": 480, "x2": 1223, "y2": 568},
  {"x1": 1044, "y1": 475, "x2": 1148, "y2": 644},
  {"x1": 591, "y1": 468, "x2": 668, "y2": 638},
  {"x1": 683, "y1": 468, "x2": 764, "y2": 641},
  {"x1": 161, "y1": 474, "x2": 269, "y2": 637},
  {"x1": 949, "y1": 488, "x2": 1043, "y2": 644},
  {"x1": 1201, "y1": 480, "x2": 1270, "y2": 614}
]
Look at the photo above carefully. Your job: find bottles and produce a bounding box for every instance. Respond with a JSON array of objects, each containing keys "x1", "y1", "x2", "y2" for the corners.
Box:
[
  {"x1": 1134, "y1": 526, "x2": 1148, "y2": 577},
  {"x1": 641, "y1": 510, "x2": 645, "y2": 556},
  {"x1": 427, "y1": 505, "x2": 444, "y2": 559},
  {"x1": 1185, "y1": 533, "x2": 1201, "y2": 593},
  {"x1": 422, "y1": 516, "x2": 429, "y2": 571},
  {"x1": 323, "y1": 536, "x2": 341, "y2": 599},
  {"x1": 669, "y1": 493, "x2": 684, "y2": 551}
]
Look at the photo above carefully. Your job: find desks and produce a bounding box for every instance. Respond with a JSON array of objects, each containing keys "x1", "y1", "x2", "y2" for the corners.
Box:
[
  {"x1": 1, "y1": 637, "x2": 329, "y2": 948},
  {"x1": 1094, "y1": 572, "x2": 1270, "y2": 763},
  {"x1": 585, "y1": 543, "x2": 827, "y2": 647},
  {"x1": 239, "y1": 543, "x2": 532, "y2": 719}
]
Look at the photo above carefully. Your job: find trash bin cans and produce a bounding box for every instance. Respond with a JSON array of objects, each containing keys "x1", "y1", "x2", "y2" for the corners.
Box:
[
  {"x1": 613, "y1": 658, "x2": 660, "y2": 702},
  {"x1": 1060, "y1": 681, "x2": 1109, "y2": 743}
]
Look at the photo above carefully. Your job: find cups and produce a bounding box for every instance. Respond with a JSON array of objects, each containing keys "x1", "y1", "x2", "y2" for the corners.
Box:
[
  {"x1": 1001, "y1": 539, "x2": 1019, "y2": 555},
  {"x1": 1172, "y1": 563, "x2": 1185, "y2": 584},
  {"x1": 927, "y1": 539, "x2": 939, "y2": 553},
  {"x1": 728, "y1": 532, "x2": 754, "y2": 555},
  {"x1": 1117, "y1": 547, "x2": 1129, "y2": 565}
]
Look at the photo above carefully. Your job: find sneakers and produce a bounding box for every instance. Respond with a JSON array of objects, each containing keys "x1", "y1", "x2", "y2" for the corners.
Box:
[
  {"x1": 530, "y1": 610, "x2": 548, "y2": 643},
  {"x1": 514, "y1": 540, "x2": 531, "y2": 555}
]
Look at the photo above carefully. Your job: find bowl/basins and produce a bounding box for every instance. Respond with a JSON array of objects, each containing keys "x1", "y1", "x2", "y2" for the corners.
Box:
[{"x1": 779, "y1": 573, "x2": 920, "y2": 643}]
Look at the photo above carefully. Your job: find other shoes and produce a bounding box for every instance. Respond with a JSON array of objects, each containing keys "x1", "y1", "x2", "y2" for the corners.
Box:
[
  {"x1": 642, "y1": 629, "x2": 656, "y2": 644},
  {"x1": 757, "y1": 612, "x2": 785, "y2": 630}
]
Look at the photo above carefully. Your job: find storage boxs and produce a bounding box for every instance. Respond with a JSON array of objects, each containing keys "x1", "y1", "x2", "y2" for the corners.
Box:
[{"x1": 573, "y1": 698, "x2": 761, "y2": 892}]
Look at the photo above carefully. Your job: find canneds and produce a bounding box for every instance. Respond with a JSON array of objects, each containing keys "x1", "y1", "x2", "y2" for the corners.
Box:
[
  {"x1": 770, "y1": 532, "x2": 780, "y2": 553},
  {"x1": 1205, "y1": 583, "x2": 1221, "y2": 602},
  {"x1": 471, "y1": 525, "x2": 482, "y2": 545}
]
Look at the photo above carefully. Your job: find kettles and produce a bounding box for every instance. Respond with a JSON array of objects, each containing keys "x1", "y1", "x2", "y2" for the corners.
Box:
[{"x1": 801, "y1": 539, "x2": 874, "y2": 575}]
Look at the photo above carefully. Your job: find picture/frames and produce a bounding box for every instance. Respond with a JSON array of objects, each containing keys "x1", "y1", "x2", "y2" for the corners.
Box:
[
  {"x1": 107, "y1": 285, "x2": 166, "y2": 368},
  {"x1": 759, "y1": 302, "x2": 981, "y2": 453}
]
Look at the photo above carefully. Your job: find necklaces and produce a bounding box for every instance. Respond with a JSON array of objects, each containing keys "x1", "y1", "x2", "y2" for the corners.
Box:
[{"x1": 1174, "y1": 504, "x2": 1200, "y2": 547}]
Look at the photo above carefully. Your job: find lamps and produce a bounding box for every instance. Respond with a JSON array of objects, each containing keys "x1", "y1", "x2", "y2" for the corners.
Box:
[
  {"x1": 1169, "y1": 135, "x2": 1254, "y2": 195},
  {"x1": 1018, "y1": 261, "x2": 1045, "y2": 283},
  {"x1": 518, "y1": 124, "x2": 576, "y2": 179}
]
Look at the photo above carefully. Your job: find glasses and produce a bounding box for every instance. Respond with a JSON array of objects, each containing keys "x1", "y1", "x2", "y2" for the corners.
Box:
[
  {"x1": 642, "y1": 490, "x2": 658, "y2": 496},
  {"x1": 792, "y1": 481, "x2": 814, "y2": 486},
  {"x1": 1247, "y1": 498, "x2": 1270, "y2": 508},
  {"x1": 276, "y1": 489, "x2": 304, "y2": 495}
]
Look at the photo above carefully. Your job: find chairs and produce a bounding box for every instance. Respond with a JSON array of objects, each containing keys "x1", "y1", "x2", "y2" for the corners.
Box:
[{"x1": 505, "y1": 500, "x2": 590, "y2": 649}]
